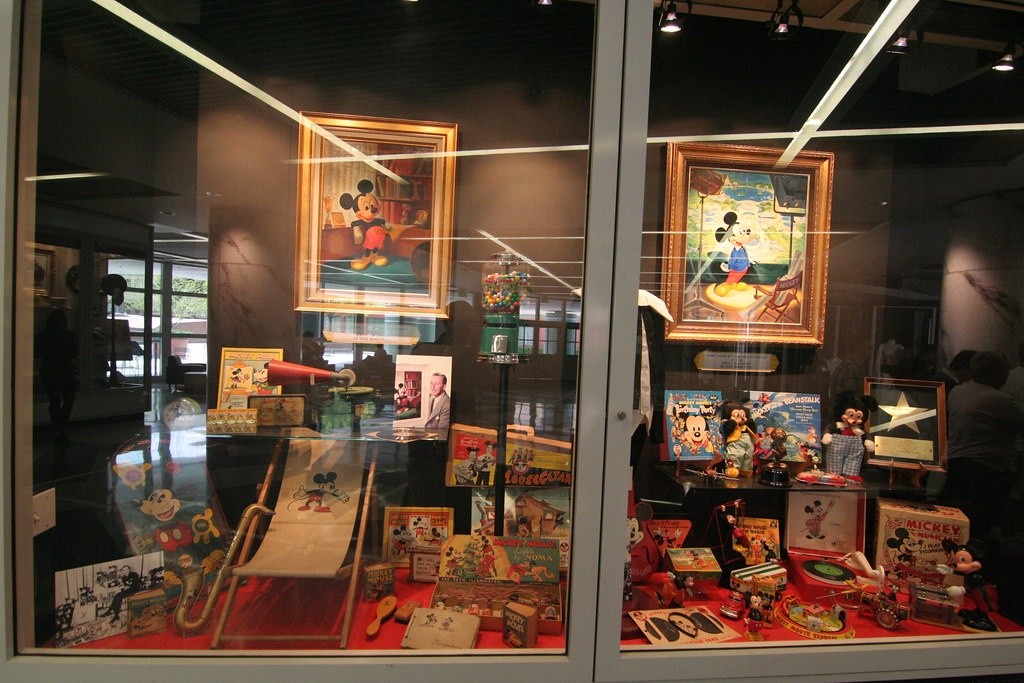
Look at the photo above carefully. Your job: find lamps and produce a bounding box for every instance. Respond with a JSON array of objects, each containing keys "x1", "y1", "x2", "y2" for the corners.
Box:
[
  {"x1": 992, "y1": 40, "x2": 1017, "y2": 72},
  {"x1": 537, "y1": 0, "x2": 553, "y2": 6},
  {"x1": 886, "y1": 21, "x2": 927, "y2": 56},
  {"x1": 764, "y1": 0, "x2": 805, "y2": 44},
  {"x1": 659, "y1": 0, "x2": 684, "y2": 34}
]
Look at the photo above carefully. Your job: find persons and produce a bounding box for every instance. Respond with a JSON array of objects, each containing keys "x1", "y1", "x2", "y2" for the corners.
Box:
[
  {"x1": 374, "y1": 343, "x2": 388, "y2": 362},
  {"x1": 875, "y1": 339, "x2": 905, "y2": 378},
  {"x1": 894, "y1": 345, "x2": 1024, "y2": 536},
  {"x1": 424, "y1": 372, "x2": 451, "y2": 429},
  {"x1": 476, "y1": 441, "x2": 496, "y2": 485},
  {"x1": 98, "y1": 565, "x2": 139, "y2": 622},
  {"x1": 301, "y1": 330, "x2": 325, "y2": 363},
  {"x1": 453, "y1": 447, "x2": 480, "y2": 486}
]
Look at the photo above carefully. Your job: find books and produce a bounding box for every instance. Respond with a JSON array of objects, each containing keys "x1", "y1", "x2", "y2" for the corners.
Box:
[{"x1": 502, "y1": 600, "x2": 539, "y2": 648}]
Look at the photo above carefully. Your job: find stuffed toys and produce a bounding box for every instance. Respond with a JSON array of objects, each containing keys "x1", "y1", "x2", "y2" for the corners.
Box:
[
  {"x1": 718, "y1": 396, "x2": 757, "y2": 478},
  {"x1": 819, "y1": 389, "x2": 879, "y2": 484}
]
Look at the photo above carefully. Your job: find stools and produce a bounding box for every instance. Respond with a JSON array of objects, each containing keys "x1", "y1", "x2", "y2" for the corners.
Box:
[{"x1": 184, "y1": 372, "x2": 207, "y2": 395}]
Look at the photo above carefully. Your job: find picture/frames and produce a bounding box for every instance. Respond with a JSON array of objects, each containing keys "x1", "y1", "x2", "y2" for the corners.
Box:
[
  {"x1": 217, "y1": 344, "x2": 286, "y2": 409},
  {"x1": 292, "y1": 107, "x2": 462, "y2": 320},
  {"x1": 864, "y1": 373, "x2": 950, "y2": 473},
  {"x1": 23, "y1": 245, "x2": 58, "y2": 301},
  {"x1": 660, "y1": 137, "x2": 840, "y2": 350}
]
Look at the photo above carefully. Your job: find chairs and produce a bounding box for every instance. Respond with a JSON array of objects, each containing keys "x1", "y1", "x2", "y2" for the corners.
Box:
[
  {"x1": 164, "y1": 354, "x2": 207, "y2": 391},
  {"x1": 197, "y1": 424, "x2": 385, "y2": 649}
]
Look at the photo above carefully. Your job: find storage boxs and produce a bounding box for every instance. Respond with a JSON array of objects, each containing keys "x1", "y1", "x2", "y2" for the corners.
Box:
[
  {"x1": 665, "y1": 545, "x2": 723, "y2": 587},
  {"x1": 407, "y1": 545, "x2": 441, "y2": 581},
  {"x1": 785, "y1": 490, "x2": 869, "y2": 604},
  {"x1": 730, "y1": 560, "x2": 790, "y2": 594},
  {"x1": 873, "y1": 496, "x2": 970, "y2": 605},
  {"x1": 245, "y1": 391, "x2": 308, "y2": 427},
  {"x1": 429, "y1": 534, "x2": 564, "y2": 633},
  {"x1": 206, "y1": 406, "x2": 258, "y2": 434}
]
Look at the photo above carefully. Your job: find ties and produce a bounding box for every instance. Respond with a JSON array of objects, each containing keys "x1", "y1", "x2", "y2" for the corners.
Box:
[{"x1": 431, "y1": 397, "x2": 435, "y2": 413}]
